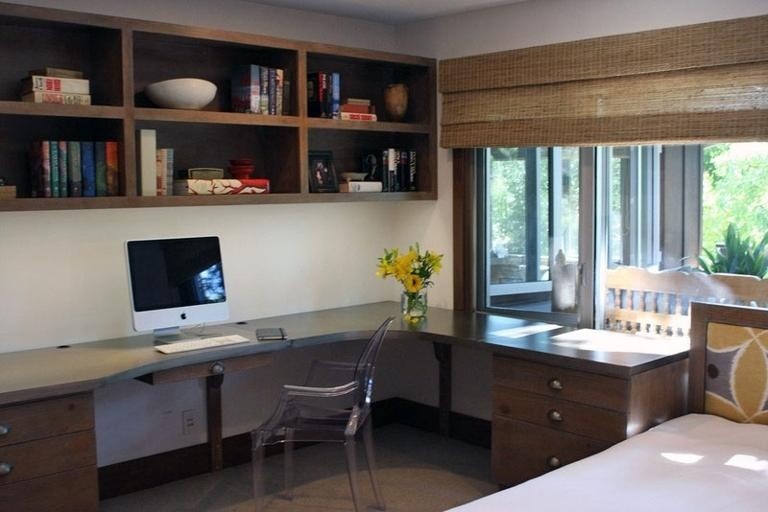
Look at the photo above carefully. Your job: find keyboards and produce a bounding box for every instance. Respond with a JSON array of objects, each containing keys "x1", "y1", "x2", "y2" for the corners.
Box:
[{"x1": 155, "y1": 334, "x2": 249, "y2": 355}]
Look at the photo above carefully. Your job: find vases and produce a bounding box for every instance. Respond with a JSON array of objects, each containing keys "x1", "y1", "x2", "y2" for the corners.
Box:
[
  {"x1": 401, "y1": 288, "x2": 428, "y2": 317},
  {"x1": 402, "y1": 317, "x2": 428, "y2": 334}
]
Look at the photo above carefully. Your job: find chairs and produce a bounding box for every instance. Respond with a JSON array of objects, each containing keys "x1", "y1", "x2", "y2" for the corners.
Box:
[{"x1": 249, "y1": 314, "x2": 396, "y2": 512}]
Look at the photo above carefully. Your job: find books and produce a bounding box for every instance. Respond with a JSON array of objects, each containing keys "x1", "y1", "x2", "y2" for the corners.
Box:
[
  {"x1": 26, "y1": 65, "x2": 85, "y2": 80},
  {"x1": 337, "y1": 180, "x2": 383, "y2": 193},
  {"x1": 35, "y1": 138, "x2": 122, "y2": 198},
  {"x1": 19, "y1": 75, "x2": 91, "y2": 96},
  {"x1": 20, "y1": 92, "x2": 92, "y2": 105},
  {"x1": 256, "y1": 327, "x2": 288, "y2": 340},
  {"x1": 138, "y1": 127, "x2": 174, "y2": 198},
  {"x1": 379, "y1": 150, "x2": 418, "y2": 195},
  {"x1": 243, "y1": 62, "x2": 378, "y2": 123}
]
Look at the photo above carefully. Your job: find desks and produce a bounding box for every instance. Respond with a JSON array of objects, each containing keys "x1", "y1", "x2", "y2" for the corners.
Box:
[{"x1": 0, "y1": 300, "x2": 691, "y2": 512}]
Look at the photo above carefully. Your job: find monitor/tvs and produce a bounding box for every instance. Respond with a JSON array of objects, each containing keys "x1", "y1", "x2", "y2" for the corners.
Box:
[{"x1": 125, "y1": 235, "x2": 229, "y2": 345}]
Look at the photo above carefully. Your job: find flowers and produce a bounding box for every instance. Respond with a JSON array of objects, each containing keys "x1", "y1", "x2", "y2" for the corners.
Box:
[{"x1": 375, "y1": 241, "x2": 446, "y2": 317}]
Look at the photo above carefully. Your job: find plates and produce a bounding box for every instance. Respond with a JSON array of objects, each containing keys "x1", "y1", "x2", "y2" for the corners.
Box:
[{"x1": 341, "y1": 172, "x2": 368, "y2": 182}]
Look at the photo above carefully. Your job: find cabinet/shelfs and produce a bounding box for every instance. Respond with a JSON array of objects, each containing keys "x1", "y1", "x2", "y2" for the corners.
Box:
[{"x1": 0, "y1": 3, "x2": 438, "y2": 211}]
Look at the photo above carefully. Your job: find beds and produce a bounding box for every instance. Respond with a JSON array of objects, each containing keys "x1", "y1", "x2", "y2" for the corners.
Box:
[{"x1": 441, "y1": 301, "x2": 768, "y2": 512}]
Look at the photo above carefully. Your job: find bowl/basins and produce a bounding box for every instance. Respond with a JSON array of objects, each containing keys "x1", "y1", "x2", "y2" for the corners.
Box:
[
  {"x1": 142, "y1": 76, "x2": 217, "y2": 111},
  {"x1": 227, "y1": 158, "x2": 255, "y2": 178}
]
